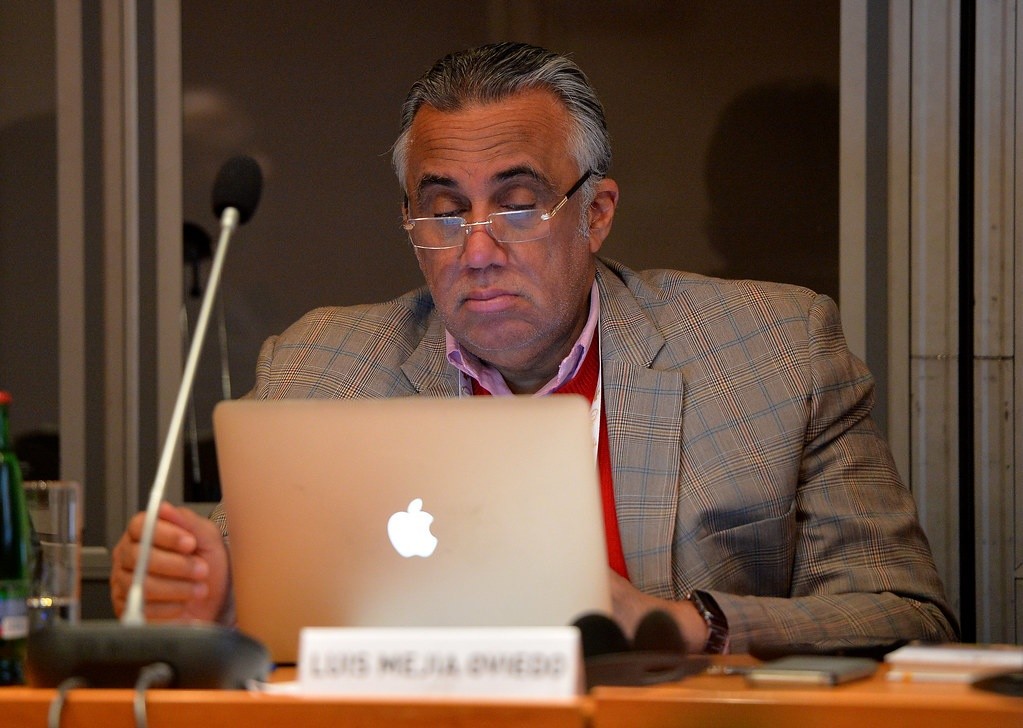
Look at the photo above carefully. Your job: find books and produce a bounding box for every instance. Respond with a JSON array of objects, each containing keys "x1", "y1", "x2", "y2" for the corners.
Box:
[
  {"x1": 884, "y1": 640, "x2": 1023, "y2": 687},
  {"x1": 745, "y1": 655, "x2": 879, "y2": 685}
]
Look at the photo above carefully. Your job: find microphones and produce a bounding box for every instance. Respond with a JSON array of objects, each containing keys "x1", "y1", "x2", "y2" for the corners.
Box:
[{"x1": 24, "y1": 157, "x2": 275, "y2": 692}]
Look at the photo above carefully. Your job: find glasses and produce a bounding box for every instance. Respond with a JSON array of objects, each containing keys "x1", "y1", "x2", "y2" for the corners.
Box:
[{"x1": 403, "y1": 169, "x2": 601, "y2": 250}]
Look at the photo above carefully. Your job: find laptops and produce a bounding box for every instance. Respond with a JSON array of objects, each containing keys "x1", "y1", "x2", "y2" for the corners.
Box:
[{"x1": 211, "y1": 397, "x2": 620, "y2": 670}]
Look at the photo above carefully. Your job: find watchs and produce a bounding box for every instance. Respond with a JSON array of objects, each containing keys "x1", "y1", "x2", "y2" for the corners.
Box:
[{"x1": 684, "y1": 587, "x2": 730, "y2": 675}]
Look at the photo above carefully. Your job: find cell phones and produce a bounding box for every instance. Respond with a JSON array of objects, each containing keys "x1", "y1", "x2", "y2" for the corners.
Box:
[{"x1": 748, "y1": 656, "x2": 878, "y2": 688}]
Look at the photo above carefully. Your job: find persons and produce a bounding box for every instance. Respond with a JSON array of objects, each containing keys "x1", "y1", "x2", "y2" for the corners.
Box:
[{"x1": 109, "y1": 39, "x2": 962, "y2": 661}]
